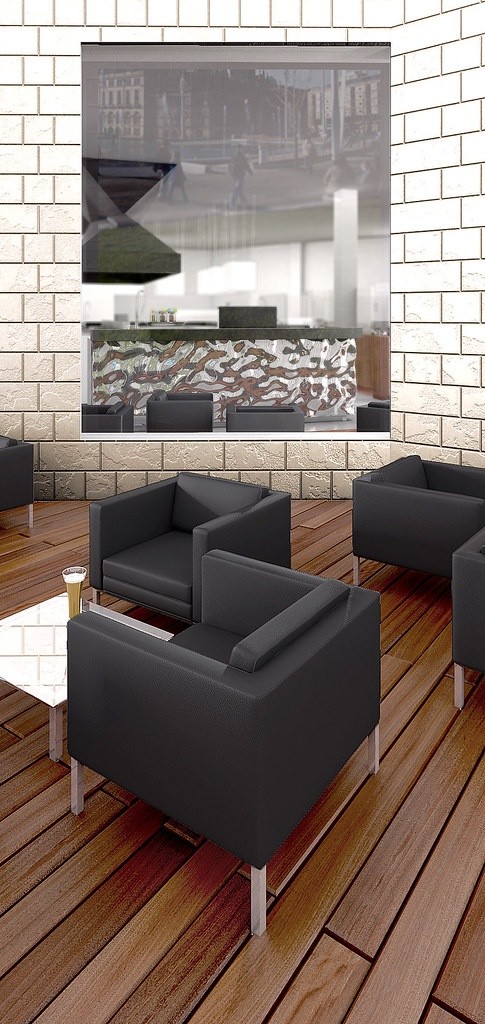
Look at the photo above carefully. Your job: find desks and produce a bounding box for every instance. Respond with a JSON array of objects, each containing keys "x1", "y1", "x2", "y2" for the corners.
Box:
[{"x1": 0, "y1": 590, "x2": 176, "y2": 816}]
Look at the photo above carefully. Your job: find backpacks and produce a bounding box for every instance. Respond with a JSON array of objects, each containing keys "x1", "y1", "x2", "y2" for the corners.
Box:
[{"x1": 229, "y1": 155, "x2": 242, "y2": 177}]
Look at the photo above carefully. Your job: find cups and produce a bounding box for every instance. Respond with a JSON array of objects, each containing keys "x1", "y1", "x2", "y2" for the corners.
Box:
[{"x1": 62, "y1": 567, "x2": 87, "y2": 618}]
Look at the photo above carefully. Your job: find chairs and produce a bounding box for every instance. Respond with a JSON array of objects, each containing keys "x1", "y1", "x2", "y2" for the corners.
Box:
[
  {"x1": 0, "y1": 433, "x2": 485, "y2": 938},
  {"x1": 82, "y1": 389, "x2": 392, "y2": 434}
]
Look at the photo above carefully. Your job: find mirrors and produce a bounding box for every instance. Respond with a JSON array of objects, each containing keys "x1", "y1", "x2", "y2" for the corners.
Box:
[{"x1": 79, "y1": 42, "x2": 394, "y2": 440}]
